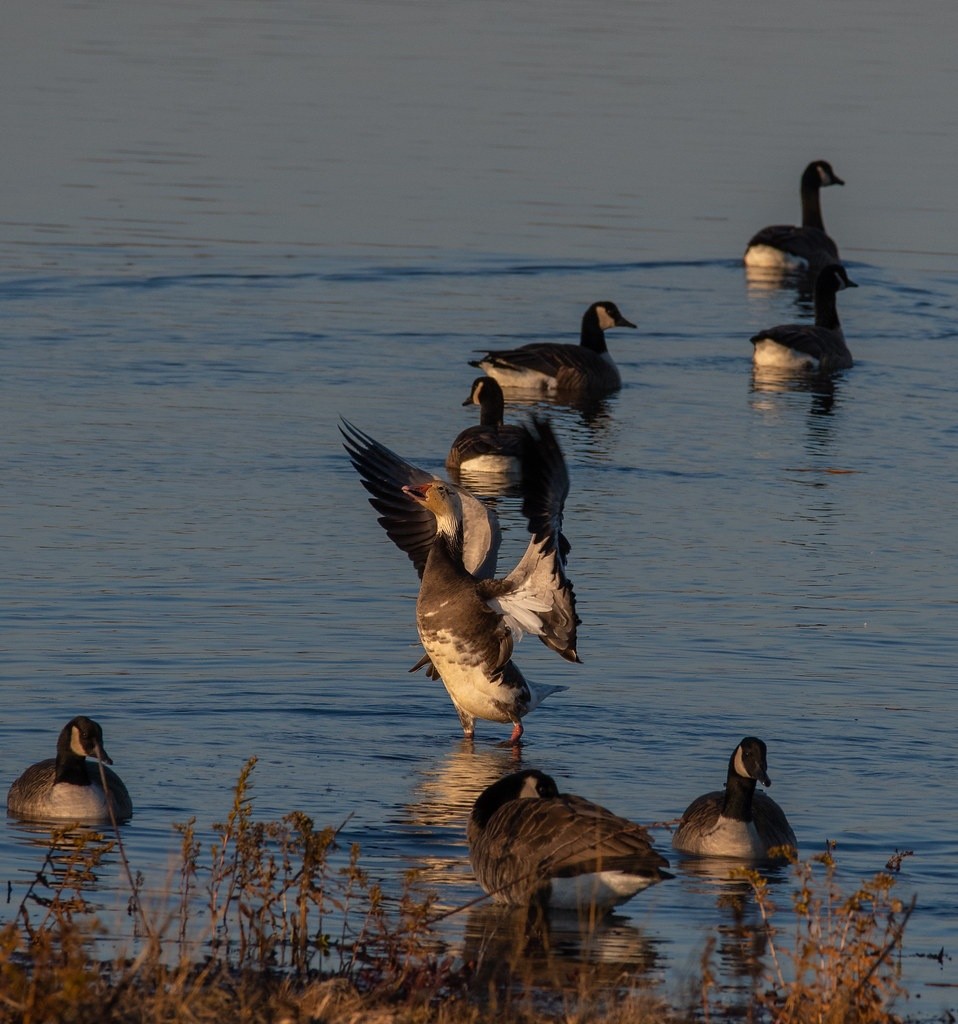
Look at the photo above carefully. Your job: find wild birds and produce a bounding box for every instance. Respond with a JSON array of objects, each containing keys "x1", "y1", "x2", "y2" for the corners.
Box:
[
  {"x1": 675, "y1": 735, "x2": 797, "y2": 857},
  {"x1": 467, "y1": 301, "x2": 639, "y2": 392},
  {"x1": 744, "y1": 160, "x2": 845, "y2": 273},
  {"x1": 7, "y1": 716, "x2": 133, "y2": 820},
  {"x1": 467, "y1": 769, "x2": 675, "y2": 909},
  {"x1": 337, "y1": 377, "x2": 581, "y2": 744},
  {"x1": 749, "y1": 264, "x2": 859, "y2": 370}
]
